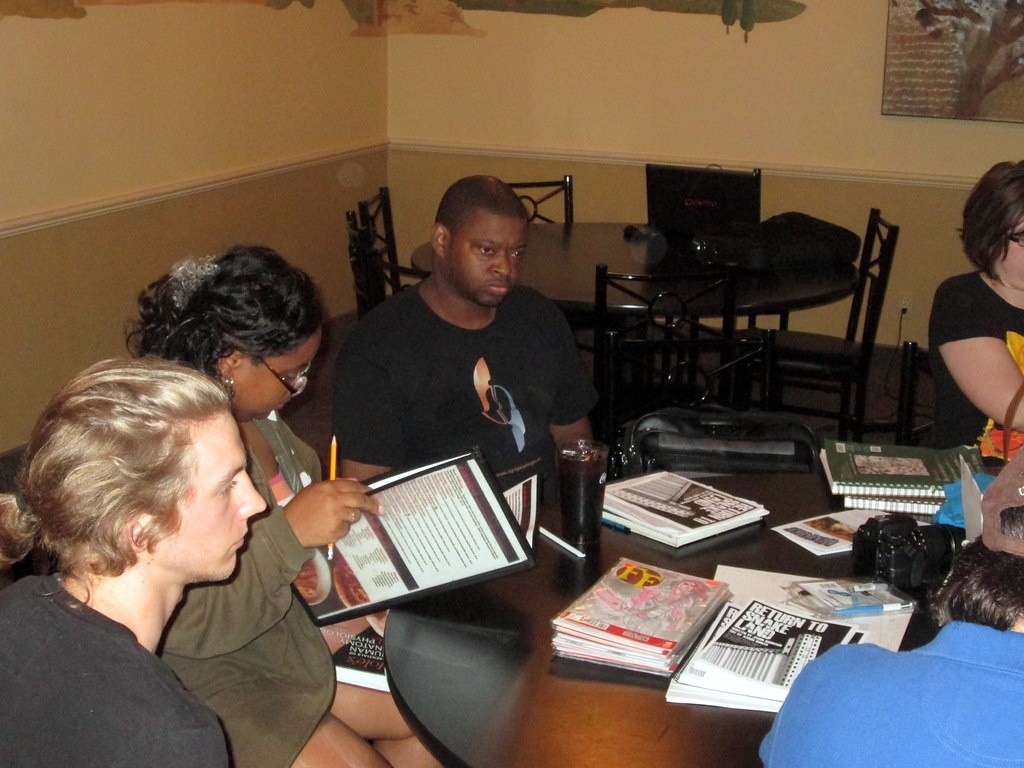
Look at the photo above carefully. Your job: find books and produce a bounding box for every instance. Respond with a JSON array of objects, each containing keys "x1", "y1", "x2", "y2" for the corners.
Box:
[
  {"x1": 664, "y1": 599, "x2": 869, "y2": 714},
  {"x1": 819, "y1": 438, "x2": 984, "y2": 515},
  {"x1": 601, "y1": 472, "x2": 770, "y2": 549},
  {"x1": 549, "y1": 558, "x2": 730, "y2": 677}
]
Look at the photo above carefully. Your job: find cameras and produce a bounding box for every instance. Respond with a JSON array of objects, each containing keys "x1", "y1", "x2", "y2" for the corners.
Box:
[{"x1": 853, "y1": 514, "x2": 966, "y2": 591}]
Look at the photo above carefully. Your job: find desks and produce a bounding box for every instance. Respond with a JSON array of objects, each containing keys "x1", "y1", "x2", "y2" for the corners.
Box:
[
  {"x1": 412, "y1": 220, "x2": 864, "y2": 415},
  {"x1": 385, "y1": 462, "x2": 1005, "y2": 767}
]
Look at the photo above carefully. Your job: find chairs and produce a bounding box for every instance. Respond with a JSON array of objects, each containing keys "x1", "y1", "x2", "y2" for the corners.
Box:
[
  {"x1": 502, "y1": 175, "x2": 576, "y2": 230},
  {"x1": 589, "y1": 208, "x2": 970, "y2": 524},
  {"x1": 345, "y1": 179, "x2": 419, "y2": 314}
]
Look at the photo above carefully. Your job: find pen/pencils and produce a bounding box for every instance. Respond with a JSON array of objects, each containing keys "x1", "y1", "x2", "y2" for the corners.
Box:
[
  {"x1": 327, "y1": 435, "x2": 336, "y2": 561},
  {"x1": 597, "y1": 512, "x2": 630, "y2": 535},
  {"x1": 538, "y1": 525, "x2": 586, "y2": 558}
]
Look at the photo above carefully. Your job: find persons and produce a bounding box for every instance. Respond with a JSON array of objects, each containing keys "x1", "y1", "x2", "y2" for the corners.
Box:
[
  {"x1": 759, "y1": 446, "x2": 1024, "y2": 768},
  {"x1": 924, "y1": 161, "x2": 1024, "y2": 450},
  {"x1": 325, "y1": 175, "x2": 610, "y2": 513},
  {"x1": 0, "y1": 357, "x2": 268, "y2": 768},
  {"x1": 119, "y1": 244, "x2": 441, "y2": 768}
]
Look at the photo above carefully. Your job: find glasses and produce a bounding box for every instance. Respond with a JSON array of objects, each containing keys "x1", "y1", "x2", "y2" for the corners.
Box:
[
  {"x1": 1006, "y1": 231, "x2": 1024, "y2": 247},
  {"x1": 256, "y1": 356, "x2": 311, "y2": 397}
]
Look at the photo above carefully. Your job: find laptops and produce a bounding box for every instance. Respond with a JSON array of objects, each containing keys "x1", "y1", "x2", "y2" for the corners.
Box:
[{"x1": 646, "y1": 163, "x2": 761, "y2": 241}]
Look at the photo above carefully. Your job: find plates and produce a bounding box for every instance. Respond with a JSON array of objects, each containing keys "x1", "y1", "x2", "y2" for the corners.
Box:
[
  {"x1": 306, "y1": 547, "x2": 332, "y2": 606},
  {"x1": 333, "y1": 567, "x2": 352, "y2": 608}
]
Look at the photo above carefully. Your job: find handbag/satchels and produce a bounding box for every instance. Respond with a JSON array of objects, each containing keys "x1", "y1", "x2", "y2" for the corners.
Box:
[
  {"x1": 612, "y1": 402, "x2": 820, "y2": 477},
  {"x1": 647, "y1": 163, "x2": 760, "y2": 243},
  {"x1": 760, "y1": 211, "x2": 861, "y2": 276}
]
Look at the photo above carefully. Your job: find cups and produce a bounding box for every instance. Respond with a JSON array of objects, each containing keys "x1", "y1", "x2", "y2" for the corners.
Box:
[{"x1": 553, "y1": 439, "x2": 610, "y2": 545}]
